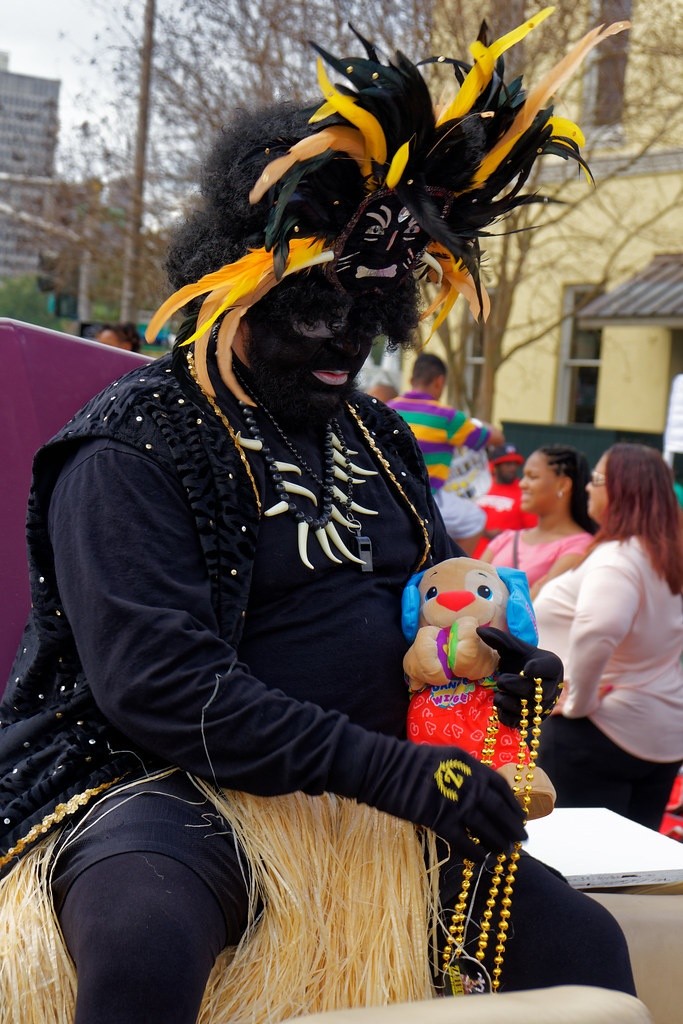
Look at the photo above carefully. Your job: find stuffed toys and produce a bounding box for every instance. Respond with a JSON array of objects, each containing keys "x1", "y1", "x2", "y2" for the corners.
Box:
[{"x1": 401, "y1": 557, "x2": 566, "y2": 822}]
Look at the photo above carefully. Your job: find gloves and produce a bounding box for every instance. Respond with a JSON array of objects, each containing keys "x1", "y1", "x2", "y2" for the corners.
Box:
[
  {"x1": 476, "y1": 626, "x2": 564, "y2": 728},
  {"x1": 331, "y1": 726, "x2": 528, "y2": 865}
]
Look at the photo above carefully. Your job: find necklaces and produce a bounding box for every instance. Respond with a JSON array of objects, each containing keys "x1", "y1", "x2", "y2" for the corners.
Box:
[
  {"x1": 440, "y1": 666, "x2": 543, "y2": 990},
  {"x1": 186, "y1": 310, "x2": 431, "y2": 597}
]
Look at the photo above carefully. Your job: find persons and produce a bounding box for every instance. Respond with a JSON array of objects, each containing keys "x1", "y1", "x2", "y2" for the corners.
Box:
[
  {"x1": 476, "y1": 446, "x2": 595, "y2": 602},
  {"x1": 93, "y1": 321, "x2": 141, "y2": 354},
  {"x1": 0, "y1": 101, "x2": 639, "y2": 1023},
  {"x1": 365, "y1": 380, "x2": 538, "y2": 561},
  {"x1": 530, "y1": 441, "x2": 683, "y2": 834},
  {"x1": 385, "y1": 354, "x2": 507, "y2": 499}
]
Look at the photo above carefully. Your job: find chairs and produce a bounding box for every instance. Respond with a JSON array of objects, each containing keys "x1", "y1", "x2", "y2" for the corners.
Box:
[{"x1": 0, "y1": 319, "x2": 683, "y2": 1023}]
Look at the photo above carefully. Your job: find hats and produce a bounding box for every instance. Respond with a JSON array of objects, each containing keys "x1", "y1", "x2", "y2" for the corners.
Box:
[{"x1": 490, "y1": 443, "x2": 525, "y2": 466}]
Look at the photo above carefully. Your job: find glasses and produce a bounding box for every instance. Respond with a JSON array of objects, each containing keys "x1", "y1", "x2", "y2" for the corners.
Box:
[{"x1": 588, "y1": 470, "x2": 608, "y2": 488}]
